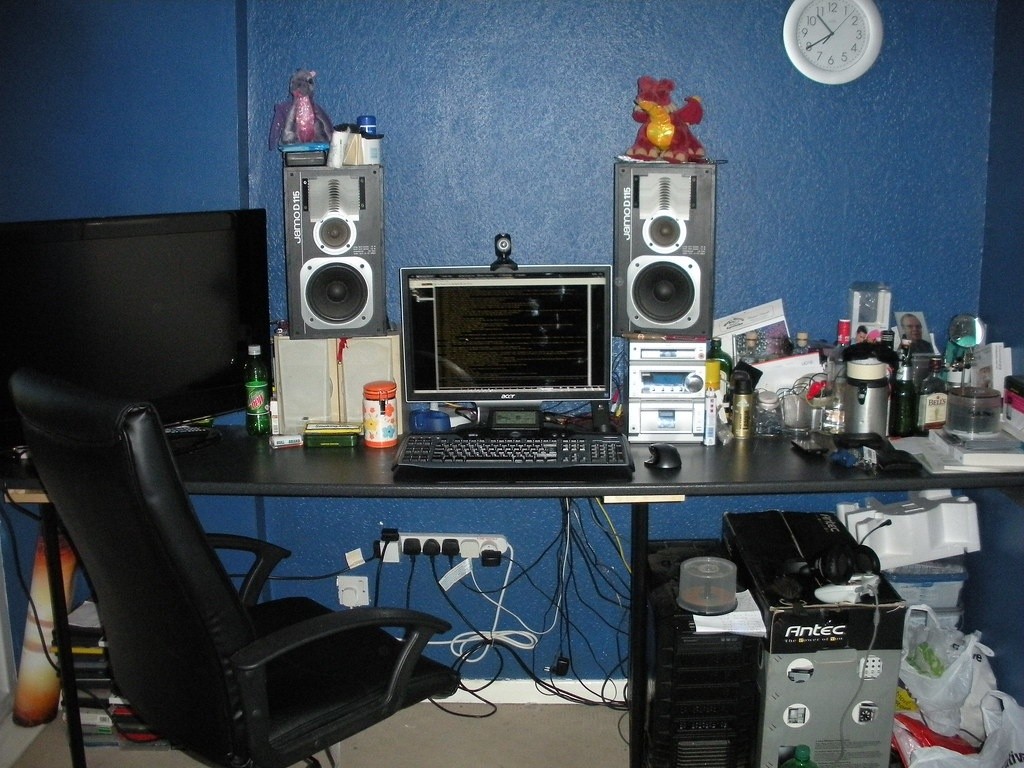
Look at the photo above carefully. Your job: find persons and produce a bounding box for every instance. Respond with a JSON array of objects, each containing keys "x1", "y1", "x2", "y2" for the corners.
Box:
[
  {"x1": 850, "y1": 325, "x2": 867, "y2": 345},
  {"x1": 899, "y1": 313, "x2": 935, "y2": 355}
]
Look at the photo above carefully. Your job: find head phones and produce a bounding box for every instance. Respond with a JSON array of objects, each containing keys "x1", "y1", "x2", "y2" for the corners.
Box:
[{"x1": 777, "y1": 540, "x2": 881, "y2": 585}]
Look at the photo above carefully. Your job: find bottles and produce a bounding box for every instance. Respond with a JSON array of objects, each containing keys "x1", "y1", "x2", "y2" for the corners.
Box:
[
  {"x1": 357, "y1": 114, "x2": 376, "y2": 134},
  {"x1": 780, "y1": 745, "x2": 818, "y2": 768},
  {"x1": 743, "y1": 330, "x2": 812, "y2": 368},
  {"x1": 707, "y1": 337, "x2": 733, "y2": 431},
  {"x1": 270, "y1": 387, "x2": 281, "y2": 434},
  {"x1": 728, "y1": 370, "x2": 753, "y2": 438},
  {"x1": 243, "y1": 345, "x2": 268, "y2": 436},
  {"x1": 889, "y1": 337, "x2": 949, "y2": 435},
  {"x1": 758, "y1": 391, "x2": 781, "y2": 436},
  {"x1": 703, "y1": 359, "x2": 720, "y2": 445},
  {"x1": 824, "y1": 319, "x2": 850, "y2": 435}
]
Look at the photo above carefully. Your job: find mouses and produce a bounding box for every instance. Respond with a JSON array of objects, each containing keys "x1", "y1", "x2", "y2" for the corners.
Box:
[{"x1": 644, "y1": 445, "x2": 686, "y2": 466}]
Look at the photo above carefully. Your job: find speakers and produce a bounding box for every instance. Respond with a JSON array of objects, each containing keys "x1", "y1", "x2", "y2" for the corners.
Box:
[
  {"x1": 283, "y1": 164, "x2": 388, "y2": 338},
  {"x1": 613, "y1": 161, "x2": 717, "y2": 340}
]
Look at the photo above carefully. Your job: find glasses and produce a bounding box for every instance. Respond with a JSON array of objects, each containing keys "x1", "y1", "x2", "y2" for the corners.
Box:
[{"x1": 745, "y1": 338, "x2": 756, "y2": 342}]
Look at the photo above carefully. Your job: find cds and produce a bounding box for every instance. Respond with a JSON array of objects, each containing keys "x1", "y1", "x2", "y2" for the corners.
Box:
[{"x1": 680, "y1": 585, "x2": 734, "y2": 611}]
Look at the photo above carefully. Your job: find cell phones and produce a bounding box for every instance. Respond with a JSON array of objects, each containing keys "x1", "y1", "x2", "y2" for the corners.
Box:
[{"x1": 792, "y1": 440, "x2": 829, "y2": 454}]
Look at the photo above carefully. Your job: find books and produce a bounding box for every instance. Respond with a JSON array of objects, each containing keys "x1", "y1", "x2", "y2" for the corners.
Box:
[{"x1": 889, "y1": 429, "x2": 1024, "y2": 474}]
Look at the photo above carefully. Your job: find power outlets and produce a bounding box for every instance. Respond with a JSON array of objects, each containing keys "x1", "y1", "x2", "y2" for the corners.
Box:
[{"x1": 335, "y1": 575, "x2": 370, "y2": 608}]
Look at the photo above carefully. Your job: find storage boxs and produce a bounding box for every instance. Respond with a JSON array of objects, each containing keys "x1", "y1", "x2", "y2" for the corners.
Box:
[{"x1": 884, "y1": 562, "x2": 968, "y2": 609}]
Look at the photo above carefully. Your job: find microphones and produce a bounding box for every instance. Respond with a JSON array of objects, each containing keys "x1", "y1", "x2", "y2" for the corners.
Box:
[{"x1": 860, "y1": 519, "x2": 892, "y2": 546}]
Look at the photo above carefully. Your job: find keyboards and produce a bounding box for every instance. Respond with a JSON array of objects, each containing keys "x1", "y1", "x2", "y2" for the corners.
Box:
[{"x1": 391, "y1": 434, "x2": 635, "y2": 481}]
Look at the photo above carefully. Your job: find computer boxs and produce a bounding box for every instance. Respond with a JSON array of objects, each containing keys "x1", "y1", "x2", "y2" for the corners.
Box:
[{"x1": 639, "y1": 539, "x2": 766, "y2": 768}]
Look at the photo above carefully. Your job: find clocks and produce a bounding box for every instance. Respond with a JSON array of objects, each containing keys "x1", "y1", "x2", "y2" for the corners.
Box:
[{"x1": 783, "y1": 0, "x2": 883, "y2": 85}]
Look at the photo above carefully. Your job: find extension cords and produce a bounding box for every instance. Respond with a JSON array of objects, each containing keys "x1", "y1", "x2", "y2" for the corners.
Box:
[{"x1": 379, "y1": 532, "x2": 509, "y2": 556}]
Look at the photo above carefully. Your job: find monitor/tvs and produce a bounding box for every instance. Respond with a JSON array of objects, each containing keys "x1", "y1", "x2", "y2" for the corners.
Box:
[
  {"x1": 0, "y1": 209, "x2": 275, "y2": 478},
  {"x1": 399, "y1": 264, "x2": 613, "y2": 441}
]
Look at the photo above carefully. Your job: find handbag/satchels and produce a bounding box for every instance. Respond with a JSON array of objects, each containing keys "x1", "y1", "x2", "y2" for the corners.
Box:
[{"x1": 892, "y1": 604, "x2": 1024, "y2": 768}]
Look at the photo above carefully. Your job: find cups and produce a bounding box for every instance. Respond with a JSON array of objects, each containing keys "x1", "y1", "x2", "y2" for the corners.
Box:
[
  {"x1": 362, "y1": 380, "x2": 399, "y2": 446},
  {"x1": 783, "y1": 395, "x2": 813, "y2": 431},
  {"x1": 844, "y1": 357, "x2": 888, "y2": 440}
]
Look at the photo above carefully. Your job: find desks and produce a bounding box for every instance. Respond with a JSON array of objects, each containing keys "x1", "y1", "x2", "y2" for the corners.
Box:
[{"x1": 0, "y1": 422, "x2": 1024, "y2": 768}]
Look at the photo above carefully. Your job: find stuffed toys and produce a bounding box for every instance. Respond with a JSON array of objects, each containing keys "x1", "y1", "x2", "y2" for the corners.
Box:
[
  {"x1": 625, "y1": 75, "x2": 708, "y2": 163},
  {"x1": 268, "y1": 69, "x2": 335, "y2": 151}
]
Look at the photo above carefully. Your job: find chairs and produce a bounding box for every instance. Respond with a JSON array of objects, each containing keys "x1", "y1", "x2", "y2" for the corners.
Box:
[{"x1": 12, "y1": 396, "x2": 461, "y2": 768}]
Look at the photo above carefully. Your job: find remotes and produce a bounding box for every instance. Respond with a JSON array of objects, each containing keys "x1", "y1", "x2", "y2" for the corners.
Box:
[{"x1": 163, "y1": 427, "x2": 209, "y2": 439}]
